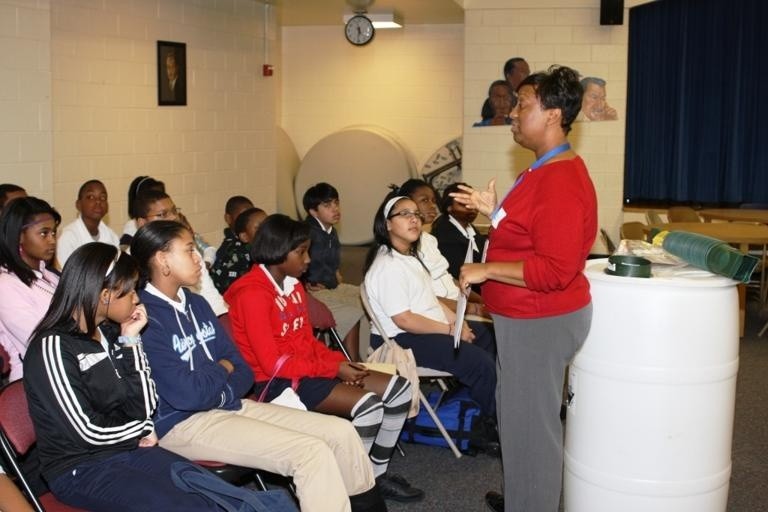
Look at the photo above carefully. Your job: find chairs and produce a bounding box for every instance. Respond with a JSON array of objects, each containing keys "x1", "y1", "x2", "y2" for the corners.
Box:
[{"x1": 0, "y1": 205, "x2": 768, "y2": 511}]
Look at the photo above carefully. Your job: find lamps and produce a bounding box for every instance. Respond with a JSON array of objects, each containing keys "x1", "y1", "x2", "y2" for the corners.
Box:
[{"x1": 341, "y1": 13, "x2": 404, "y2": 30}]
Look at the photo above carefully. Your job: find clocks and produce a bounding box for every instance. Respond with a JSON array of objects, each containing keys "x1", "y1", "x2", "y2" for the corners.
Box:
[{"x1": 345, "y1": 16, "x2": 373, "y2": 45}]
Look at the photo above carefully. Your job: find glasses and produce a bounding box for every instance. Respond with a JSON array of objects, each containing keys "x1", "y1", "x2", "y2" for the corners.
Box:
[
  {"x1": 388, "y1": 210, "x2": 423, "y2": 219},
  {"x1": 145, "y1": 208, "x2": 180, "y2": 218}
]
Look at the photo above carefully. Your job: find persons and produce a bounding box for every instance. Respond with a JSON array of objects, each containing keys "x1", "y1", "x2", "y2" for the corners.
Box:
[
  {"x1": 471, "y1": 58, "x2": 617, "y2": 128},
  {"x1": 1, "y1": 176, "x2": 567, "y2": 511},
  {"x1": 158, "y1": 51, "x2": 183, "y2": 102},
  {"x1": 448, "y1": 64, "x2": 598, "y2": 511}
]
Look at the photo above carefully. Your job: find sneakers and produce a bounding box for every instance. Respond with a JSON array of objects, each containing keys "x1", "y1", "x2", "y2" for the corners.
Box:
[
  {"x1": 474, "y1": 428, "x2": 501, "y2": 457},
  {"x1": 485, "y1": 490, "x2": 505, "y2": 511},
  {"x1": 377, "y1": 475, "x2": 425, "y2": 501}
]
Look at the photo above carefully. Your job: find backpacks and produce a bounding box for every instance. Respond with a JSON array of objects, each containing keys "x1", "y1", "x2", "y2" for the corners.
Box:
[{"x1": 400, "y1": 388, "x2": 481, "y2": 458}]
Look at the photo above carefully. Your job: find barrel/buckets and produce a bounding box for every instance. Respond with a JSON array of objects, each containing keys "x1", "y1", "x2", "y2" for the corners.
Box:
[{"x1": 561, "y1": 255, "x2": 741, "y2": 512}]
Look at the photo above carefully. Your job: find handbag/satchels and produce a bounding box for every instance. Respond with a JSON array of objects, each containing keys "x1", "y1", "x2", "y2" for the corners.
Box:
[
  {"x1": 250, "y1": 354, "x2": 307, "y2": 409},
  {"x1": 171, "y1": 461, "x2": 299, "y2": 512}
]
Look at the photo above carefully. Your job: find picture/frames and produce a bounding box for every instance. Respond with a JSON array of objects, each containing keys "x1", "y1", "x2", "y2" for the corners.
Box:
[{"x1": 156, "y1": 39, "x2": 188, "y2": 105}]
